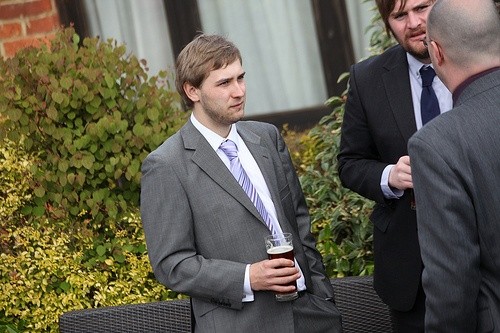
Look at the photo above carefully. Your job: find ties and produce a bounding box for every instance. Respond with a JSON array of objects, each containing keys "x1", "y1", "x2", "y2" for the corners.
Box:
[
  {"x1": 419, "y1": 66, "x2": 441, "y2": 126},
  {"x1": 219, "y1": 139, "x2": 281, "y2": 247}
]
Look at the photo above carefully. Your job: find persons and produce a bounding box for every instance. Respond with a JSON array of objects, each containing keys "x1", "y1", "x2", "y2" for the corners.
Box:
[
  {"x1": 335, "y1": 0, "x2": 452, "y2": 333},
  {"x1": 140, "y1": 34, "x2": 342, "y2": 333},
  {"x1": 407, "y1": 0, "x2": 500, "y2": 333}
]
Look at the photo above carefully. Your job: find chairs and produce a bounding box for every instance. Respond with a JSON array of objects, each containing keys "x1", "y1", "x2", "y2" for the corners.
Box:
[
  {"x1": 329, "y1": 276, "x2": 394, "y2": 333},
  {"x1": 58, "y1": 297, "x2": 192, "y2": 333}
]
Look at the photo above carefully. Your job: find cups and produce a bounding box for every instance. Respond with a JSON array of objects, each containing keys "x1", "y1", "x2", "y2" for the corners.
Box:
[
  {"x1": 264, "y1": 233, "x2": 298, "y2": 302},
  {"x1": 410, "y1": 188, "x2": 416, "y2": 210}
]
